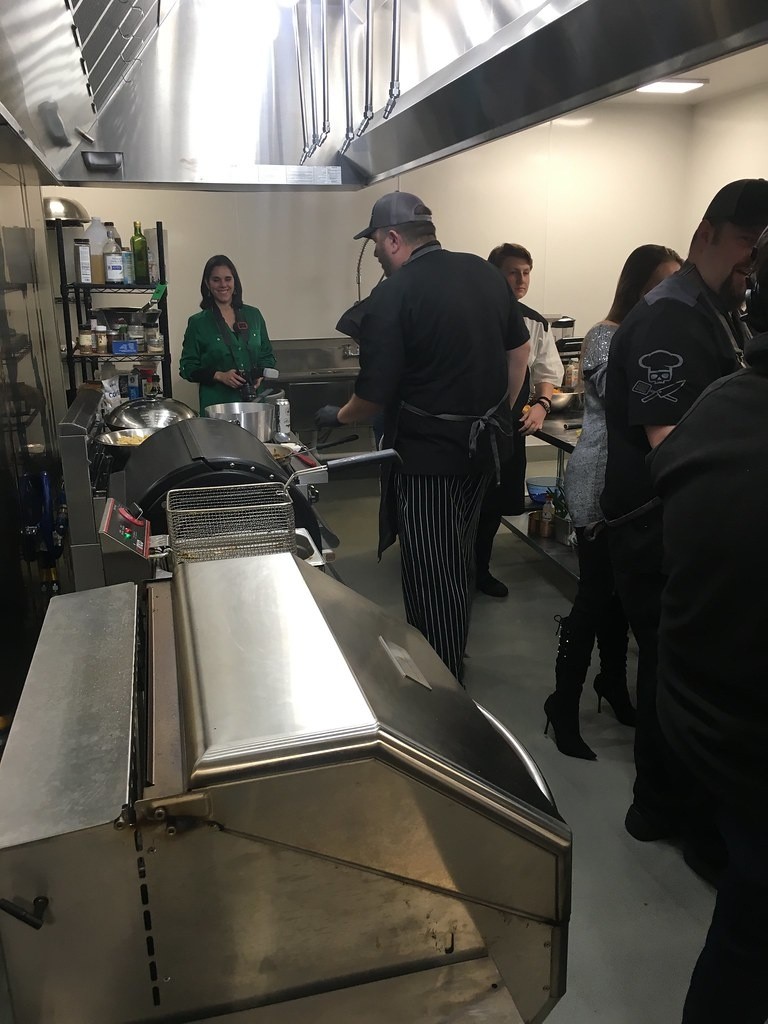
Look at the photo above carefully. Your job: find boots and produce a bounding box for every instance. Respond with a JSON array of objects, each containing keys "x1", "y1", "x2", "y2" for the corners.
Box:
[
  {"x1": 594, "y1": 624, "x2": 637, "y2": 727},
  {"x1": 544, "y1": 615, "x2": 597, "y2": 760}
]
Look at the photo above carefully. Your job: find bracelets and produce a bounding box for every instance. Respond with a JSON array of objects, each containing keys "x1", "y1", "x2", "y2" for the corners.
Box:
[{"x1": 535, "y1": 396, "x2": 551, "y2": 414}]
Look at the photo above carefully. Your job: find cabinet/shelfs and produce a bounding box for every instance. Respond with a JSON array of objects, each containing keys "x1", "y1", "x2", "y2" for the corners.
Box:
[
  {"x1": 53, "y1": 218, "x2": 173, "y2": 400},
  {"x1": 0, "y1": 246, "x2": 55, "y2": 487}
]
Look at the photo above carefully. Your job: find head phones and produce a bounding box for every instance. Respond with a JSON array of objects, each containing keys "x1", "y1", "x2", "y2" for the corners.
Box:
[{"x1": 736, "y1": 224, "x2": 768, "y2": 333}]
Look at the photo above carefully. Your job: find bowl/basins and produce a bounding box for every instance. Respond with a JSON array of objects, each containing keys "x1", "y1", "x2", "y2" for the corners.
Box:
[
  {"x1": 526, "y1": 476, "x2": 563, "y2": 502},
  {"x1": 551, "y1": 393, "x2": 578, "y2": 411},
  {"x1": 528, "y1": 512, "x2": 554, "y2": 536},
  {"x1": 42, "y1": 198, "x2": 92, "y2": 229}
]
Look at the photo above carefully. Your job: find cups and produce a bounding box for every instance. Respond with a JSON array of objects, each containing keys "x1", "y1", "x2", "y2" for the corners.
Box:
[{"x1": 146, "y1": 334, "x2": 164, "y2": 355}]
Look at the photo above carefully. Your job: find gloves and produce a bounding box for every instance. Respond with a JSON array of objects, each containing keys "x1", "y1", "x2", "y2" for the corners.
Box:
[{"x1": 314, "y1": 405, "x2": 345, "y2": 430}]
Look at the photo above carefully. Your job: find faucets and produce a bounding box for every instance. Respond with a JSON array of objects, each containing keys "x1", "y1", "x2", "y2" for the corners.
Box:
[
  {"x1": 327, "y1": 370, "x2": 337, "y2": 374},
  {"x1": 341, "y1": 343, "x2": 360, "y2": 360},
  {"x1": 307, "y1": 368, "x2": 321, "y2": 376}
]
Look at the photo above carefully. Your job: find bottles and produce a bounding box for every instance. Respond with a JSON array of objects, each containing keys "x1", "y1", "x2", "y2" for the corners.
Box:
[
  {"x1": 74, "y1": 239, "x2": 92, "y2": 284},
  {"x1": 104, "y1": 222, "x2": 121, "y2": 250},
  {"x1": 129, "y1": 221, "x2": 151, "y2": 286},
  {"x1": 80, "y1": 217, "x2": 108, "y2": 284},
  {"x1": 540, "y1": 498, "x2": 555, "y2": 538},
  {"x1": 102, "y1": 231, "x2": 124, "y2": 284},
  {"x1": 147, "y1": 247, "x2": 156, "y2": 284},
  {"x1": 78, "y1": 317, "x2": 158, "y2": 355}
]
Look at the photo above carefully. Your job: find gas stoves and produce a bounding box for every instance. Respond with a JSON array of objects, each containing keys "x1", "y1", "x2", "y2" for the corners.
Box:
[{"x1": 58, "y1": 389, "x2": 339, "y2": 583}]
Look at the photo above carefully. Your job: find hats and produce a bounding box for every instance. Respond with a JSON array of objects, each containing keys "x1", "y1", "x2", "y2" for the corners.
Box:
[
  {"x1": 353, "y1": 190, "x2": 432, "y2": 240},
  {"x1": 702, "y1": 178, "x2": 768, "y2": 228}
]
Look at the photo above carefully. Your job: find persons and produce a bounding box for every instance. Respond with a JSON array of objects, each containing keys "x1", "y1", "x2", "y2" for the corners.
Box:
[
  {"x1": 316, "y1": 190, "x2": 528, "y2": 692},
  {"x1": 472, "y1": 243, "x2": 565, "y2": 599},
  {"x1": 543, "y1": 180, "x2": 768, "y2": 1024},
  {"x1": 178, "y1": 254, "x2": 278, "y2": 418}
]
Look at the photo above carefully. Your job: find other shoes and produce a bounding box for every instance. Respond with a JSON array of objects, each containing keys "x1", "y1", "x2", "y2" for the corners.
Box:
[
  {"x1": 625, "y1": 797, "x2": 689, "y2": 841},
  {"x1": 475, "y1": 573, "x2": 508, "y2": 597}
]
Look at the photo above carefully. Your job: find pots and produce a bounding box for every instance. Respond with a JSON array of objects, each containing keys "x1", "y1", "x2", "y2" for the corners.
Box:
[{"x1": 95, "y1": 388, "x2": 294, "y2": 469}]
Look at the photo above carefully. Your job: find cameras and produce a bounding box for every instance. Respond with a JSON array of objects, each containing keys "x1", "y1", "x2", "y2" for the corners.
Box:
[{"x1": 237, "y1": 366, "x2": 279, "y2": 402}]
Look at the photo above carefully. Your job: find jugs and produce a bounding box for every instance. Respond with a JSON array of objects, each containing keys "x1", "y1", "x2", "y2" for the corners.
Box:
[{"x1": 550, "y1": 315, "x2": 576, "y2": 342}]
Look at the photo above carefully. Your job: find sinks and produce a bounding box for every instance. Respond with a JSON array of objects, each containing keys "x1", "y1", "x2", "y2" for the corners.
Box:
[{"x1": 274, "y1": 374, "x2": 360, "y2": 379}]
